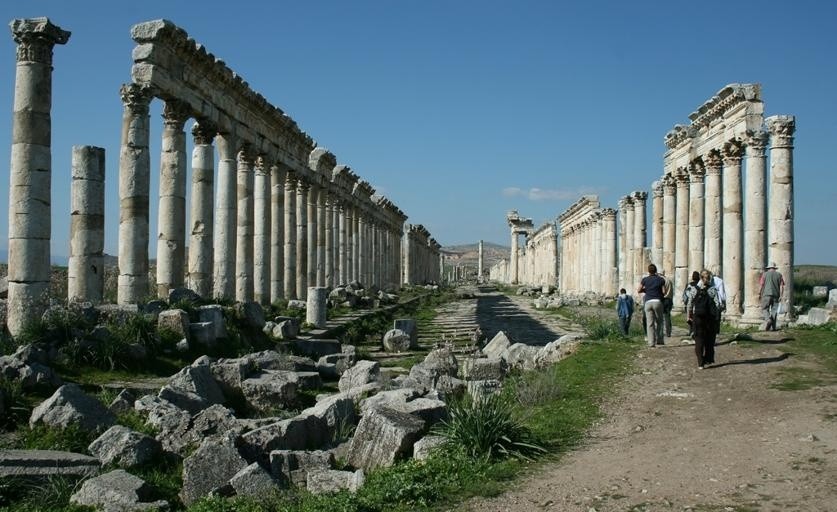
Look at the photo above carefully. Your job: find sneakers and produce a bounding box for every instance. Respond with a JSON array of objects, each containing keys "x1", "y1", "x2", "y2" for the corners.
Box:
[
  {"x1": 766, "y1": 319, "x2": 776, "y2": 331},
  {"x1": 697, "y1": 365, "x2": 704, "y2": 370}
]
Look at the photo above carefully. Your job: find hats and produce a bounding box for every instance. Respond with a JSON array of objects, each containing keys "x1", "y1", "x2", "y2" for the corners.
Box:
[{"x1": 765, "y1": 261, "x2": 778, "y2": 269}]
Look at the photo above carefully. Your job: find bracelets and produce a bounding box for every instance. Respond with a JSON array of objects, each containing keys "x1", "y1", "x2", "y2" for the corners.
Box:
[{"x1": 758, "y1": 292, "x2": 760, "y2": 295}]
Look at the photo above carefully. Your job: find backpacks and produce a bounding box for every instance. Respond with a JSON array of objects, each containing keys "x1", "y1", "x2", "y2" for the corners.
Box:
[{"x1": 694, "y1": 285, "x2": 715, "y2": 326}]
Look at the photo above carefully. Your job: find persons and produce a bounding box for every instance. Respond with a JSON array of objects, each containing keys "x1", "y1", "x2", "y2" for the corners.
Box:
[
  {"x1": 682, "y1": 271, "x2": 700, "y2": 343},
  {"x1": 657, "y1": 268, "x2": 673, "y2": 336},
  {"x1": 636, "y1": 264, "x2": 666, "y2": 347},
  {"x1": 686, "y1": 270, "x2": 723, "y2": 369},
  {"x1": 639, "y1": 293, "x2": 647, "y2": 341},
  {"x1": 758, "y1": 261, "x2": 785, "y2": 331},
  {"x1": 615, "y1": 287, "x2": 633, "y2": 336},
  {"x1": 696, "y1": 275, "x2": 727, "y2": 335}
]
